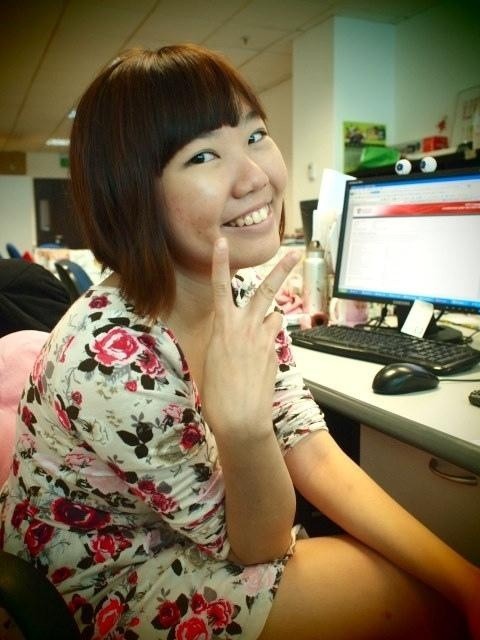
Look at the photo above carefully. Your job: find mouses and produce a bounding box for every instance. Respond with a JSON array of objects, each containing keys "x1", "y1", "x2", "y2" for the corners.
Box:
[{"x1": 371, "y1": 361, "x2": 441, "y2": 395}]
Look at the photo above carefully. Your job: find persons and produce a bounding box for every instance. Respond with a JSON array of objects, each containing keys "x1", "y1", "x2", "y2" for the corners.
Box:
[{"x1": 0, "y1": 45, "x2": 480, "y2": 639}]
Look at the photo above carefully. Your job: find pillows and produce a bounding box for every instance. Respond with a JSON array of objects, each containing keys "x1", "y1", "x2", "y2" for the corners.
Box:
[{"x1": 0, "y1": 328, "x2": 52, "y2": 490}]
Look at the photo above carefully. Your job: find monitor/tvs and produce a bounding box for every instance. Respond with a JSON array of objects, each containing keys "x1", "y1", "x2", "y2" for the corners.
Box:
[{"x1": 331, "y1": 165, "x2": 480, "y2": 341}]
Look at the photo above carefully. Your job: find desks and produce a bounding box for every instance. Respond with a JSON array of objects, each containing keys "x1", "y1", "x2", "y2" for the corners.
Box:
[{"x1": 285, "y1": 342, "x2": 480, "y2": 569}]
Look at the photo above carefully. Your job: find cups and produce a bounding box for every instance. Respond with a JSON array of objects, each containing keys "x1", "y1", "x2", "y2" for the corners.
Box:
[{"x1": 302, "y1": 239, "x2": 329, "y2": 314}]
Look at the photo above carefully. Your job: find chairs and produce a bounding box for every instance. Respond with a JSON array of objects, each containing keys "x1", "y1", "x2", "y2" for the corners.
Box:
[
  {"x1": 5, "y1": 241, "x2": 22, "y2": 259},
  {"x1": 0, "y1": 259, "x2": 83, "y2": 640},
  {"x1": 54, "y1": 258, "x2": 94, "y2": 305}
]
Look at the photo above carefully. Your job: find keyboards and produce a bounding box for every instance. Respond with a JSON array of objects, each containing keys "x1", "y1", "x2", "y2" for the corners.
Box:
[{"x1": 287, "y1": 323, "x2": 480, "y2": 377}]
